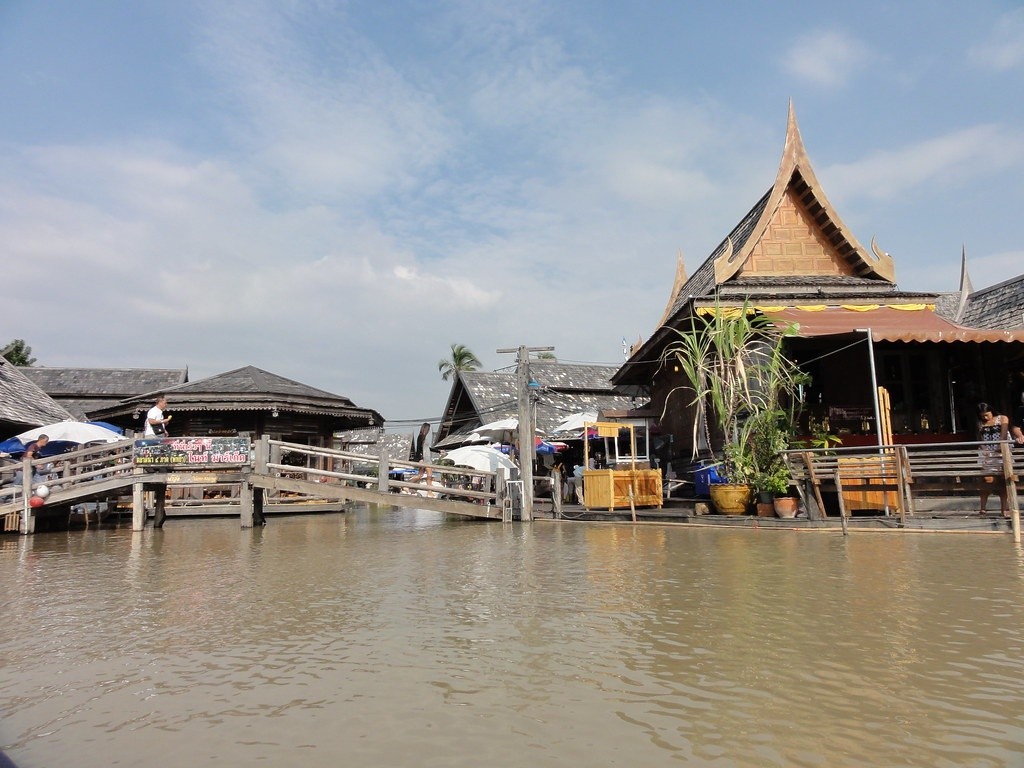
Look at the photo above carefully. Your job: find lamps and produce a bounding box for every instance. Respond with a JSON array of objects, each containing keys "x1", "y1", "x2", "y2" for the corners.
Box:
[
  {"x1": 272, "y1": 409, "x2": 280, "y2": 417},
  {"x1": 369, "y1": 419, "x2": 374, "y2": 425},
  {"x1": 133, "y1": 412, "x2": 140, "y2": 419}
]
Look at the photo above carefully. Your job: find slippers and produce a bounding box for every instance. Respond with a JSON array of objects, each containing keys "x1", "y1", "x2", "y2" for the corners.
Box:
[
  {"x1": 401, "y1": 489, "x2": 411, "y2": 495},
  {"x1": 427, "y1": 494, "x2": 436, "y2": 498}
]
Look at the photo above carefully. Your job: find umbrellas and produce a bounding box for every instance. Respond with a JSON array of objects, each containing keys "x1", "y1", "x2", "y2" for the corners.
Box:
[
  {"x1": 389, "y1": 468, "x2": 418, "y2": 474},
  {"x1": 492, "y1": 439, "x2": 556, "y2": 454},
  {"x1": 443, "y1": 445, "x2": 520, "y2": 479},
  {"x1": 466, "y1": 430, "x2": 518, "y2": 452},
  {"x1": 468, "y1": 417, "x2": 545, "y2": 460},
  {"x1": 0, "y1": 421, "x2": 127, "y2": 457},
  {"x1": 549, "y1": 412, "x2": 598, "y2": 434}
]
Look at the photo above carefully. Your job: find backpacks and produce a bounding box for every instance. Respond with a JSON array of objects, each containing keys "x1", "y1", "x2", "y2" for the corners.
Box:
[{"x1": 551, "y1": 463, "x2": 562, "y2": 479}]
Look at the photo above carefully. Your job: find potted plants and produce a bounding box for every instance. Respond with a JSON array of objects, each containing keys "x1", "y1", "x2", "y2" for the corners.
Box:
[{"x1": 649, "y1": 281, "x2": 844, "y2": 520}]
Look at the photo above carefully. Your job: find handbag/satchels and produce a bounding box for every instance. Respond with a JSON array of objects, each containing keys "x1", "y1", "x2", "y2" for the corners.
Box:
[
  {"x1": 31, "y1": 451, "x2": 41, "y2": 476},
  {"x1": 151, "y1": 423, "x2": 165, "y2": 435}
]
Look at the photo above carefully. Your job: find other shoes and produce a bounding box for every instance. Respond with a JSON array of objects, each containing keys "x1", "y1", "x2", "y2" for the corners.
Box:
[
  {"x1": 980, "y1": 512, "x2": 986, "y2": 518},
  {"x1": 1001, "y1": 509, "x2": 1009, "y2": 516}
]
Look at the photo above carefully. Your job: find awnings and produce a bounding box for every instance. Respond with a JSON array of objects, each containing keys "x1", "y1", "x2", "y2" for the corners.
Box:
[{"x1": 762, "y1": 309, "x2": 1024, "y2": 344}]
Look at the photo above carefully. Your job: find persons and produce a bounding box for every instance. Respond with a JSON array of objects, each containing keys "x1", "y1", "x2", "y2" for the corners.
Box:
[
  {"x1": 975, "y1": 403, "x2": 1014, "y2": 518},
  {"x1": 146, "y1": 396, "x2": 170, "y2": 438},
  {"x1": 402, "y1": 422, "x2": 447, "y2": 498},
  {"x1": 1010, "y1": 393, "x2": 1024, "y2": 470},
  {"x1": 15, "y1": 434, "x2": 49, "y2": 485}
]
